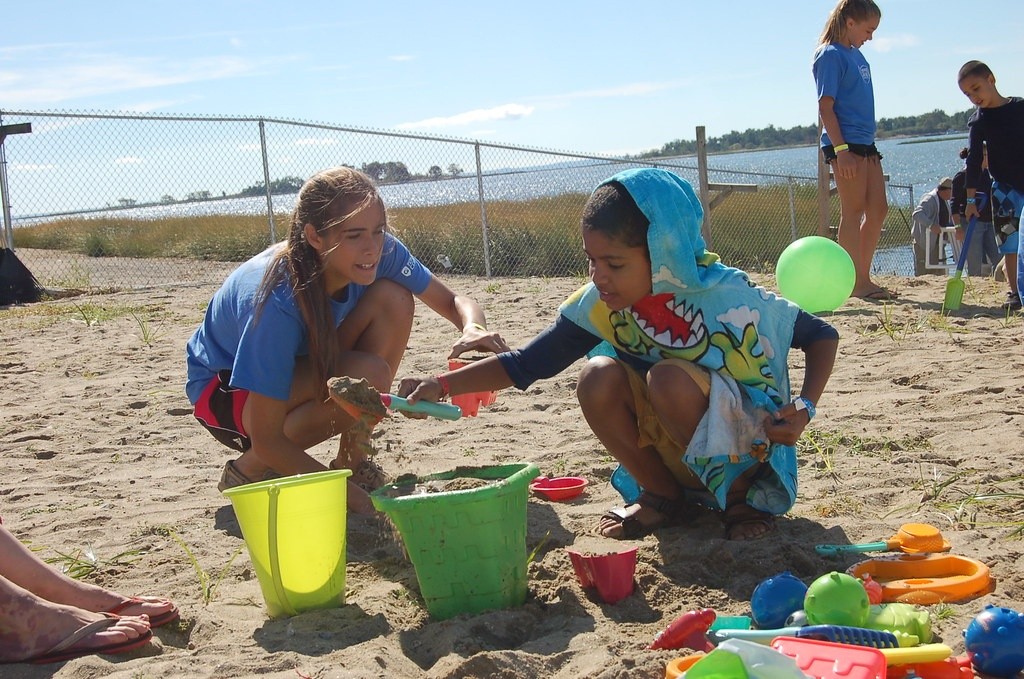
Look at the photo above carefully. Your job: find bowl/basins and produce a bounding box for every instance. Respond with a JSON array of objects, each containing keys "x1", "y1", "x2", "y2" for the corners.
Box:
[{"x1": 531, "y1": 476, "x2": 586, "y2": 498}]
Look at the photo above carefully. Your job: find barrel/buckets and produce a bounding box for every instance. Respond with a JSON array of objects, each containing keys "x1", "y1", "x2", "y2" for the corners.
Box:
[
  {"x1": 220, "y1": 468, "x2": 353, "y2": 620},
  {"x1": 370, "y1": 463, "x2": 541, "y2": 618}
]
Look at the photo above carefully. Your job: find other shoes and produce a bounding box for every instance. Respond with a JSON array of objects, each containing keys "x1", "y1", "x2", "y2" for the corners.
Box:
[
  {"x1": 1001, "y1": 290, "x2": 1021, "y2": 310},
  {"x1": 218, "y1": 459, "x2": 285, "y2": 493},
  {"x1": 329, "y1": 454, "x2": 393, "y2": 492}
]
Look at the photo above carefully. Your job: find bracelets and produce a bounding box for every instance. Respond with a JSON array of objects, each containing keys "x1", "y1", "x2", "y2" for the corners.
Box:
[
  {"x1": 967, "y1": 198, "x2": 975, "y2": 204},
  {"x1": 435, "y1": 374, "x2": 450, "y2": 402},
  {"x1": 954, "y1": 224, "x2": 962, "y2": 229},
  {"x1": 463, "y1": 323, "x2": 489, "y2": 334},
  {"x1": 793, "y1": 397, "x2": 815, "y2": 419},
  {"x1": 929, "y1": 224, "x2": 932, "y2": 229},
  {"x1": 833, "y1": 144, "x2": 849, "y2": 155}
]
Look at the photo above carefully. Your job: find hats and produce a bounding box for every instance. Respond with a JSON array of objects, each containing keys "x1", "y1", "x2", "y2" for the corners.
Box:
[{"x1": 939, "y1": 176, "x2": 953, "y2": 188}]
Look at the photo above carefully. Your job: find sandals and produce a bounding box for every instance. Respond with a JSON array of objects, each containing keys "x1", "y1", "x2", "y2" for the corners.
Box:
[
  {"x1": 721, "y1": 490, "x2": 778, "y2": 542},
  {"x1": 592, "y1": 488, "x2": 697, "y2": 540}
]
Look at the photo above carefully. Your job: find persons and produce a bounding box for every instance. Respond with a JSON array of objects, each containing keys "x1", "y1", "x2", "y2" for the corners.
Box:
[
  {"x1": 911, "y1": 174, "x2": 953, "y2": 276},
  {"x1": 185, "y1": 166, "x2": 511, "y2": 522},
  {"x1": 812, "y1": 0, "x2": 899, "y2": 301},
  {"x1": 397, "y1": 167, "x2": 840, "y2": 536},
  {"x1": 1, "y1": 516, "x2": 178, "y2": 665},
  {"x1": 951, "y1": 60, "x2": 1024, "y2": 311}
]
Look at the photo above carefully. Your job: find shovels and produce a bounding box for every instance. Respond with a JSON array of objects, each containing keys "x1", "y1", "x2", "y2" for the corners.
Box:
[
  {"x1": 944, "y1": 192, "x2": 989, "y2": 310},
  {"x1": 816, "y1": 522, "x2": 952, "y2": 555},
  {"x1": 327, "y1": 376, "x2": 461, "y2": 428}
]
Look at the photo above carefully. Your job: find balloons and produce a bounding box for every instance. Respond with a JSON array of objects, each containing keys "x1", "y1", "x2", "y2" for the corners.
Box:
[{"x1": 776, "y1": 235, "x2": 855, "y2": 314}]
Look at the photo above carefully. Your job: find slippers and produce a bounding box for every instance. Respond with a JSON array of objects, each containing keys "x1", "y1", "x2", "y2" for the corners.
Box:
[
  {"x1": 107, "y1": 592, "x2": 179, "y2": 628},
  {"x1": 27, "y1": 612, "x2": 153, "y2": 662},
  {"x1": 865, "y1": 287, "x2": 898, "y2": 300}
]
